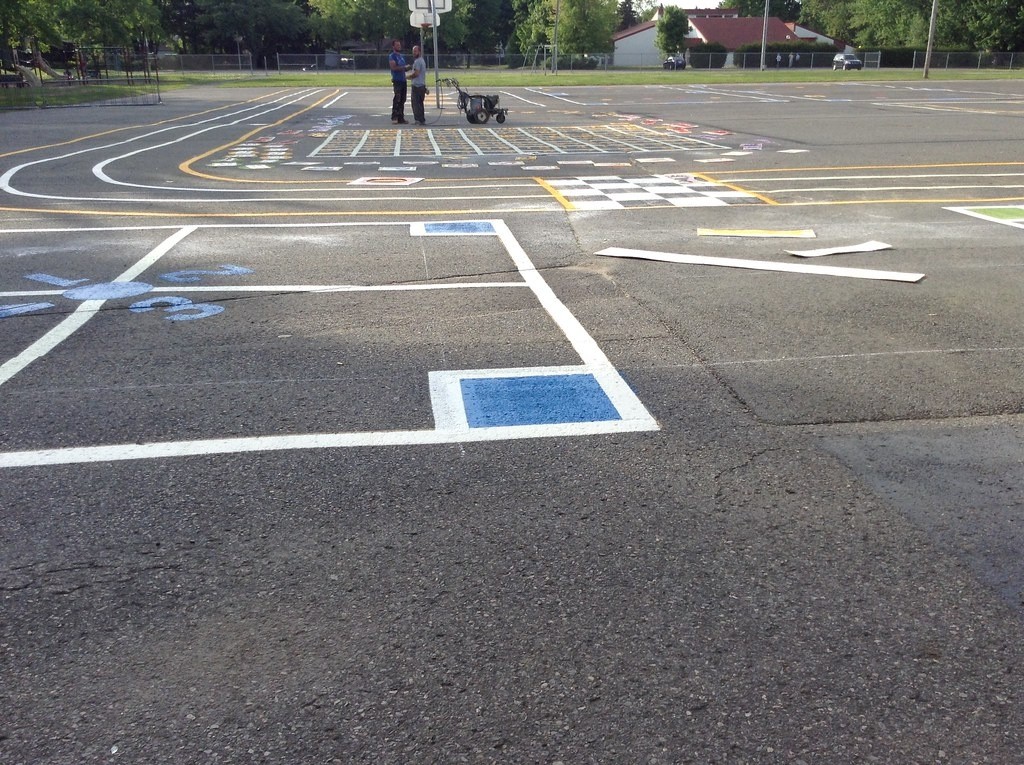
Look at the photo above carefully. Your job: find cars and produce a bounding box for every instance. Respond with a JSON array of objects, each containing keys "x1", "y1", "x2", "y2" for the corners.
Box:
[{"x1": 663, "y1": 55, "x2": 687, "y2": 70}]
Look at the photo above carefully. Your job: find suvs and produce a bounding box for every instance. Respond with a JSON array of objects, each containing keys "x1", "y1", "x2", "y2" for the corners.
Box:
[{"x1": 831, "y1": 54, "x2": 863, "y2": 71}]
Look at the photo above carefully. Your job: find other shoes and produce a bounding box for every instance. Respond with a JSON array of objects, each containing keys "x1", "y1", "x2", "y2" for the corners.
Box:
[
  {"x1": 392, "y1": 117, "x2": 400, "y2": 124},
  {"x1": 398, "y1": 118, "x2": 411, "y2": 124}
]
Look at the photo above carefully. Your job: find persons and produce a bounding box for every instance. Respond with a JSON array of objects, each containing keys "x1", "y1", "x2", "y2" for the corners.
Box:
[
  {"x1": 406, "y1": 46, "x2": 427, "y2": 126},
  {"x1": 388, "y1": 40, "x2": 411, "y2": 125},
  {"x1": 775, "y1": 53, "x2": 800, "y2": 68}
]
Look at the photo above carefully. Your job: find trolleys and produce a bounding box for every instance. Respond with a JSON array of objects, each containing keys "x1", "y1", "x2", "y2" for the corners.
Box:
[{"x1": 435, "y1": 76, "x2": 509, "y2": 125}]
